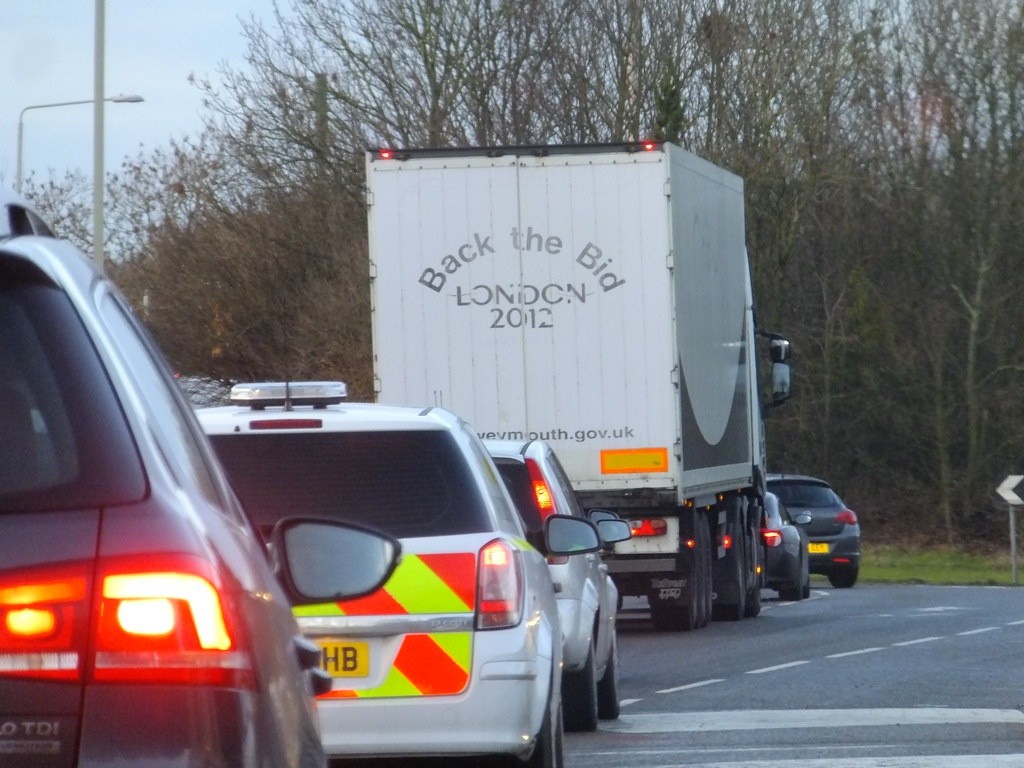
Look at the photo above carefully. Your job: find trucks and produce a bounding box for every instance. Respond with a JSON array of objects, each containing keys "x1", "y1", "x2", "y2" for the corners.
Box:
[{"x1": 363, "y1": 142, "x2": 788, "y2": 631}]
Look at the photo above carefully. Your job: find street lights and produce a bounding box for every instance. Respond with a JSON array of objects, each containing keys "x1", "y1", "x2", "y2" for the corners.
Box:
[{"x1": 15, "y1": 95, "x2": 144, "y2": 197}]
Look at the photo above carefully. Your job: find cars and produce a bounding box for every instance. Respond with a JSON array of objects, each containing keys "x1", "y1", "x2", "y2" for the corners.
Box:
[
  {"x1": 189, "y1": 380, "x2": 598, "y2": 767},
  {"x1": 760, "y1": 475, "x2": 859, "y2": 601},
  {"x1": 479, "y1": 438, "x2": 630, "y2": 735}
]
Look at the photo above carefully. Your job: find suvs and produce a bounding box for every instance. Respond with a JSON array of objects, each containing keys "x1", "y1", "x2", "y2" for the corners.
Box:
[{"x1": 1, "y1": 188, "x2": 327, "y2": 768}]
